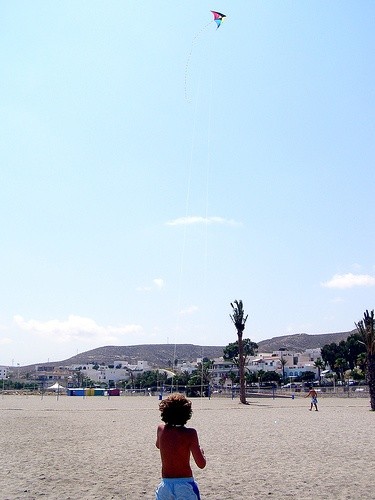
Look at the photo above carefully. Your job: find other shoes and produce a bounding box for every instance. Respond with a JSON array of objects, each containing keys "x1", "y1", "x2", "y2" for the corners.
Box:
[
  {"x1": 315, "y1": 410, "x2": 318, "y2": 411},
  {"x1": 309, "y1": 409, "x2": 312, "y2": 410}
]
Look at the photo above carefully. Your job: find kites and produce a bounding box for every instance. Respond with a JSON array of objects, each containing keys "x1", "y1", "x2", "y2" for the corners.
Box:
[{"x1": 209, "y1": 9, "x2": 227, "y2": 31}]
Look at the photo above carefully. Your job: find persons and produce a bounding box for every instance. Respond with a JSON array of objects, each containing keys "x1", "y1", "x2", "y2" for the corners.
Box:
[
  {"x1": 155, "y1": 392, "x2": 207, "y2": 500},
  {"x1": 304, "y1": 386, "x2": 318, "y2": 412}
]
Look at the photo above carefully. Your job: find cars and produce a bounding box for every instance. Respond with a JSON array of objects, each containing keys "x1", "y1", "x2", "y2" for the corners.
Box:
[
  {"x1": 283, "y1": 382, "x2": 298, "y2": 388},
  {"x1": 347, "y1": 381, "x2": 355, "y2": 386}
]
